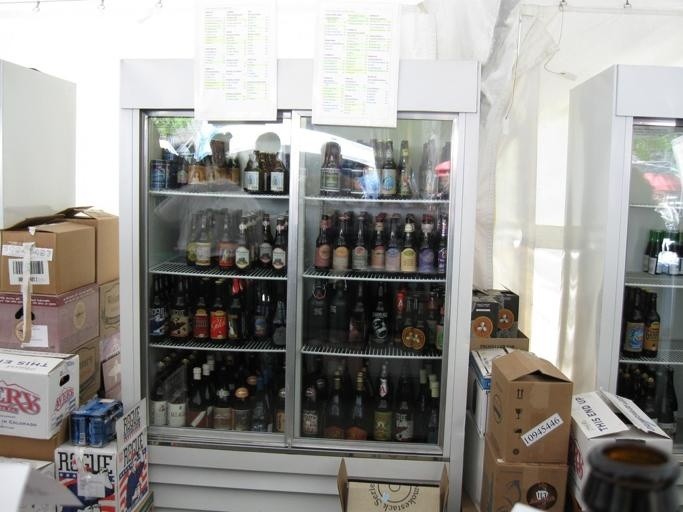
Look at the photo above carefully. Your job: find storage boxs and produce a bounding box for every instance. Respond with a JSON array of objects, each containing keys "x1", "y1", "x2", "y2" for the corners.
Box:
[{"x1": 336, "y1": 458, "x2": 450, "y2": 512}]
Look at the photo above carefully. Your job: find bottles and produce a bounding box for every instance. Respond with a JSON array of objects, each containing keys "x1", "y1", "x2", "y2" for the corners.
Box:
[
  {"x1": 619, "y1": 284, "x2": 660, "y2": 359},
  {"x1": 306, "y1": 282, "x2": 442, "y2": 348},
  {"x1": 185, "y1": 206, "x2": 287, "y2": 273},
  {"x1": 305, "y1": 360, "x2": 439, "y2": 445},
  {"x1": 244, "y1": 148, "x2": 290, "y2": 195},
  {"x1": 616, "y1": 361, "x2": 677, "y2": 437},
  {"x1": 313, "y1": 213, "x2": 448, "y2": 274},
  {"x1": 321, "y1": 134, "x2": 450, "y2": 200},
  {"x1": 151, "y1": 352, "x2": 269, "y2": 433},
  {"x1": 150, "y1": 274, "x2": 287, "y2": 347},
  {"x1": 641, "y1": 228, "x2": 682, "y2": 277}
]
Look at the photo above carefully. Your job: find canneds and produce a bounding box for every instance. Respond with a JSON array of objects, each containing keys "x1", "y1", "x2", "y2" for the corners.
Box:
[{"x1": 70, "y1": 397, "x2": 122, "y2": 448}]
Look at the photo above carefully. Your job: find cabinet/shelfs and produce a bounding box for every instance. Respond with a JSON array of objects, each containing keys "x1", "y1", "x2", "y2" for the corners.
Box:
[{"x1": 558, "y1": 65, "x2": 682, "y2": 508}]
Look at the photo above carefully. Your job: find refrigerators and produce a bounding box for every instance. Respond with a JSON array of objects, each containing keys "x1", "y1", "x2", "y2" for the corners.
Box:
[{"x1": 551, "y1": 66, "x2": 682, "y2": 438}]
